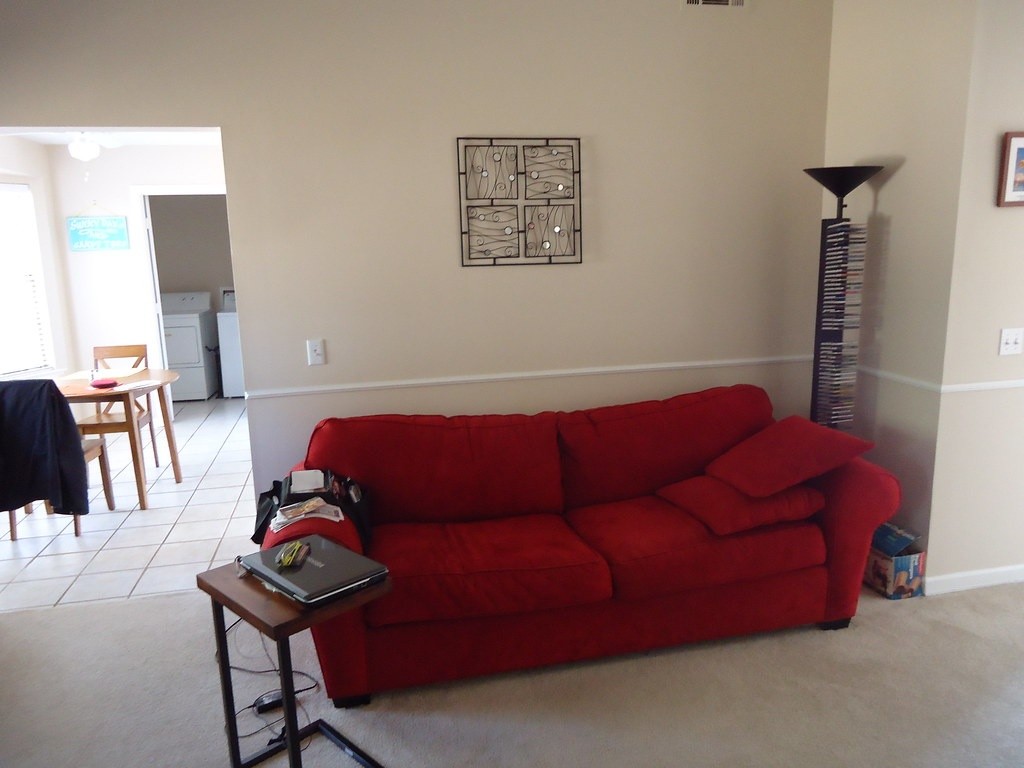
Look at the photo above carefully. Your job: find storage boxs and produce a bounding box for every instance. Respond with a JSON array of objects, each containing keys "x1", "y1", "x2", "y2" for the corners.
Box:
[{"x1": 863, "y1": 520, "x2": 927, "y2": 600}]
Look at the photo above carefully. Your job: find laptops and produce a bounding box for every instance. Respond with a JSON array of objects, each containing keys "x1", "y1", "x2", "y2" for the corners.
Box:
[{"x1": 238, "y1": 533, "x2": 390, "y2": 609}]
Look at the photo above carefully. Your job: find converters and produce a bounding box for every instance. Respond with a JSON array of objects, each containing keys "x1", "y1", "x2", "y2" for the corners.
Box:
[{"x1": 256, "y1": 692, "x2": 283, "y2": 714}]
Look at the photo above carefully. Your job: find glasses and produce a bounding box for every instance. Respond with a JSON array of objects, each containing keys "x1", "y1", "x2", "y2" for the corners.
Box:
[{"x1": 281, "y1": 540, "x2": 303, "y2": 567}]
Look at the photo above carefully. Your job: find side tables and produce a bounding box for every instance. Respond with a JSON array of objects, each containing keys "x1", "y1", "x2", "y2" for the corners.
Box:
[{"x1": 198, "y1": 537, "x2": 393, "y2": 768}]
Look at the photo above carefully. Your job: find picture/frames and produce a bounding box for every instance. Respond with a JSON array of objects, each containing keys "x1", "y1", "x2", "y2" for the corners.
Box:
[{"x1": 996, "y1": 131, "x2": 1024, "y2": 207}]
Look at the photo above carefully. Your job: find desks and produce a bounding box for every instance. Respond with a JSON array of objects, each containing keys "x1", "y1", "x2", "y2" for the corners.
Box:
[{"x1": 53, "y1": 369, "x2": 183, "y2": 510}]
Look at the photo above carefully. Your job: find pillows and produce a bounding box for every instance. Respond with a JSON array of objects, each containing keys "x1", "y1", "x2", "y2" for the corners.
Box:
[
  {"x1": 654, "y1": 475, "x2": 827, "y2": 536},
  {"x1": 707, "y1": 414, "x2": 877, "y2": 498}
]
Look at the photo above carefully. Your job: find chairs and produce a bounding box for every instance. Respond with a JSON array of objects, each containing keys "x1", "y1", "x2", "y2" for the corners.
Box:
[
  {"x1": 75, "y1": 345, "x2": 159, "y2": 488},
  {"x1": 0, "y1": 379, "x2": 115, "y2": 541}
]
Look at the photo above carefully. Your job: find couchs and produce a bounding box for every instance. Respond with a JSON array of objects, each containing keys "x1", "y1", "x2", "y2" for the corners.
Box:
[{"x1": 260, "y1": 382, "x2": 901, "y2": 709}]
